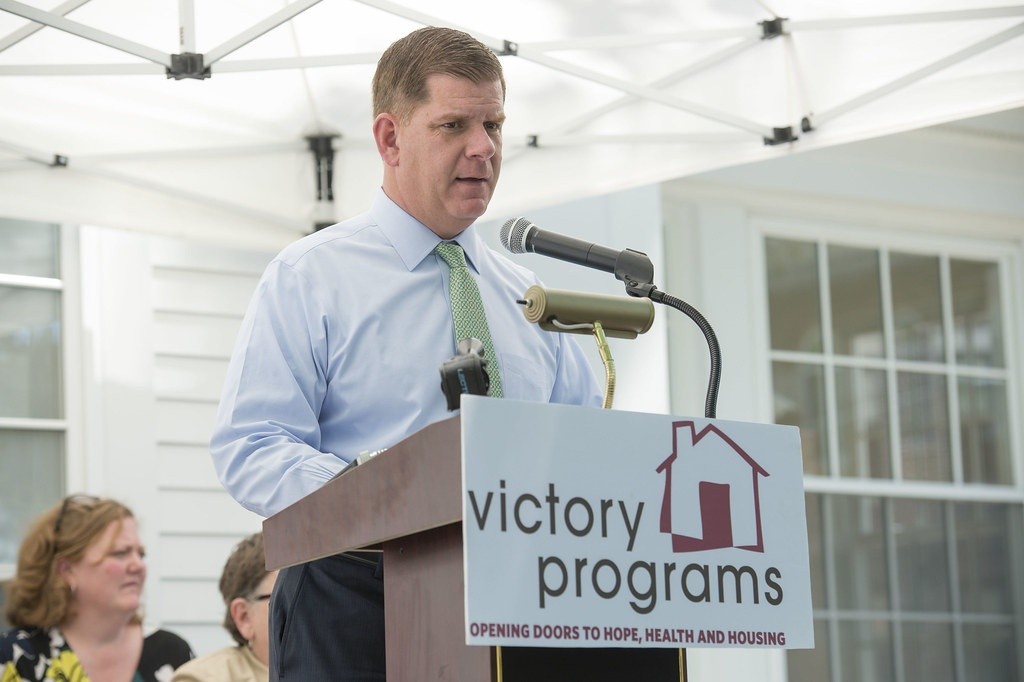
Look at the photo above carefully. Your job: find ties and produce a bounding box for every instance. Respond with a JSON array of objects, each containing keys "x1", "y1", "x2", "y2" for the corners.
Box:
[{"x1": 431, "y1": 242, "x2": 503, "y2": 399}]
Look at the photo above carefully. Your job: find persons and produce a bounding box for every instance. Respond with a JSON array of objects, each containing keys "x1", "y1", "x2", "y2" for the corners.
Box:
[
  {"x1": 0, "y1": 494, "x2": 198, "y2": 682},
  {"x1": 208, "y1": 26, "x2": 604, "y2": 682},
  {"x1": 170, "y1": 531, "x2": 280, "y2": 682}
]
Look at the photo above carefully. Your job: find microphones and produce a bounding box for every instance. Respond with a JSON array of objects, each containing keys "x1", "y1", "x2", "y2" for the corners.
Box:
[
  {"x1": 501, "y1": 217, "x2": 653, "y2": 283},
  {"x1": 439, "y1": 338, "x2": 490, "y2": 412}
]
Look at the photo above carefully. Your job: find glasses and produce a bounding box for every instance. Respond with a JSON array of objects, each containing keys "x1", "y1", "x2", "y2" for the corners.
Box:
[{"x1": 52, "y1": 493, "x2": 100, "y2": 554}]
